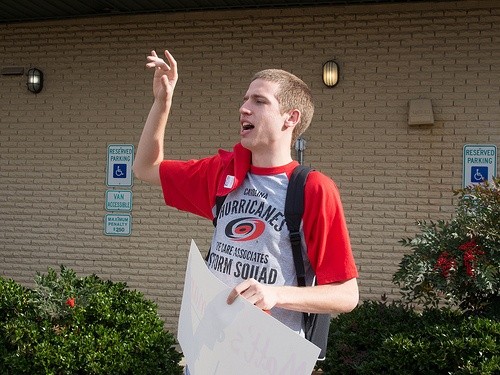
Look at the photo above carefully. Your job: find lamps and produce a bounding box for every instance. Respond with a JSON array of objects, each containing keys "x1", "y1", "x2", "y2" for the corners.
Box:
[
  {"x1": 26, "y1": 68, "x2": 43, "y2": 94},
  {"x1": 321, "y1": 61, "x2": 339, "y2": 88}
]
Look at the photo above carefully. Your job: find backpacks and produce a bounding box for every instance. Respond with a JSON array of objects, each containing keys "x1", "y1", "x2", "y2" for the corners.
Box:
[{"x1": 212, "y1": 164, "x2": 332, "y2": 375}]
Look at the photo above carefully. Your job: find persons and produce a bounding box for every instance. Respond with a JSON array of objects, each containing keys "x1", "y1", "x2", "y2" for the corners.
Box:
[{"x1": 133, "y1": 50, "x2": 360, "y2": 375}]
